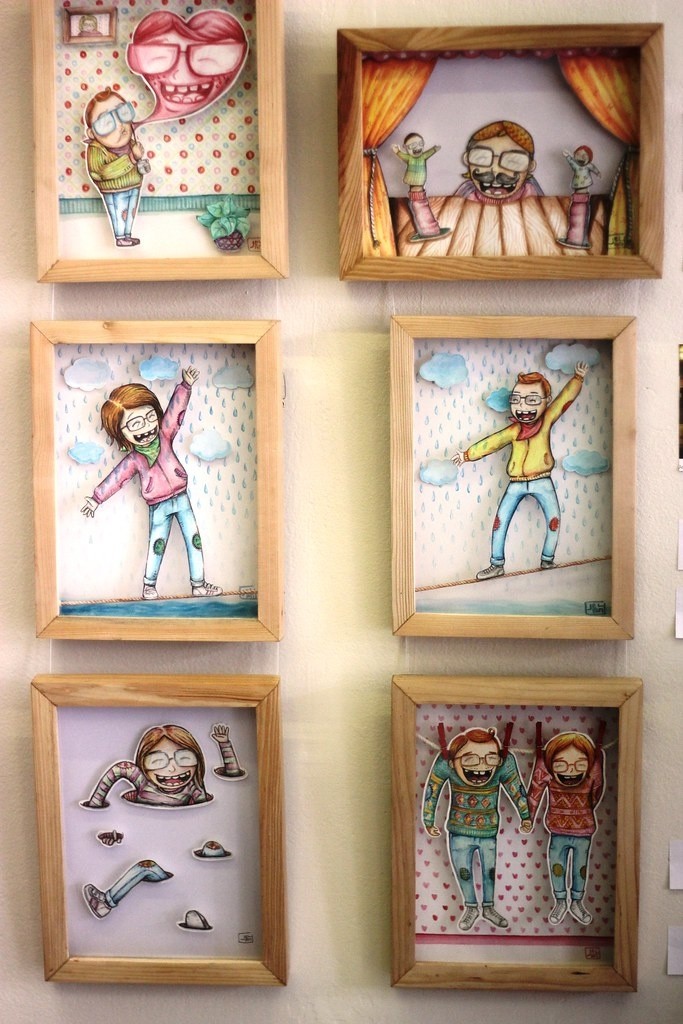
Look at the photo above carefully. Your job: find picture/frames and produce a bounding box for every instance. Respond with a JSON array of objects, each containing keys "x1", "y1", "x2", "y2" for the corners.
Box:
[
  {"x1": 31, "y1": 671, "x2": 289, "y2": 990},
  {"x1": 28, "y1": 321, "x2": 283, "y2": 643},
  {"x1": 34, "y1": 0, "x2": 289, "y2": 283},
  {"x1": 391, "y1": 673, "x2": 646, "y2": 992},
  {"x1": 334, "y1": 23, "x2": 666, "y2": 279},
  {"x1": 391, "y1": 314, "x2": 638, "y2": 644}
]
